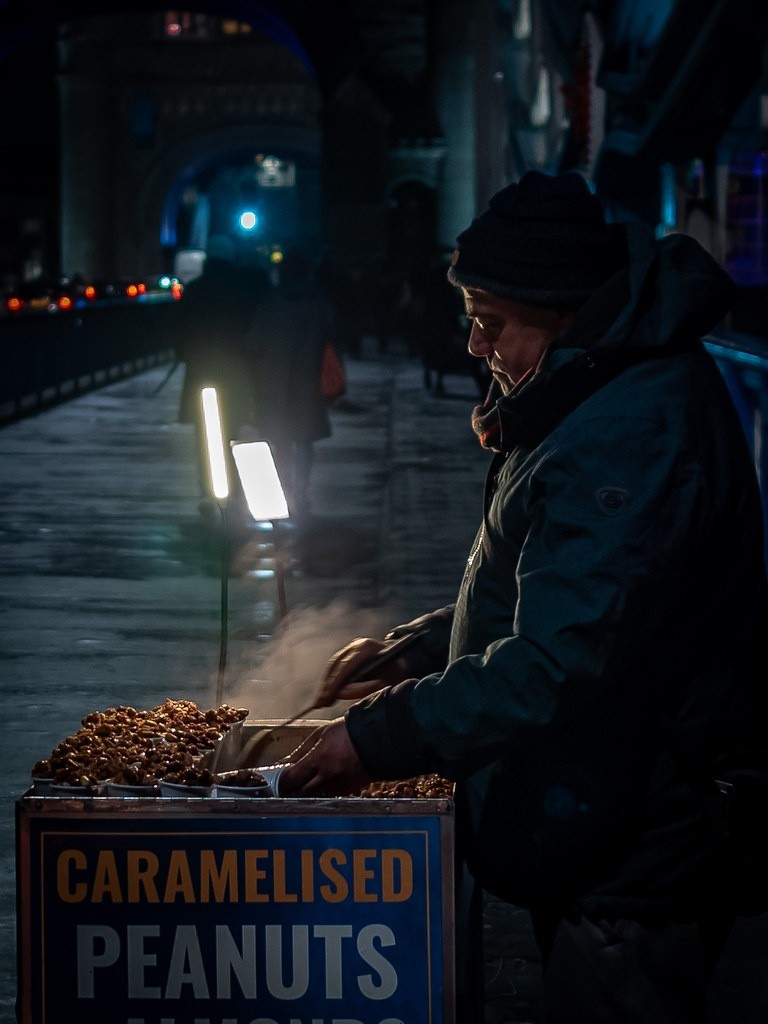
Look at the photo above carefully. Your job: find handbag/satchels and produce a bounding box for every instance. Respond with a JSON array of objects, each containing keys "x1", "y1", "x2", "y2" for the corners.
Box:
[{"x1": 319, "y1": 341, "x2": 347, "y2": 404}]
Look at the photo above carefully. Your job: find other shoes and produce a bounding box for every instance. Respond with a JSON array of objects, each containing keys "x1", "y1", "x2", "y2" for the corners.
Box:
[
  {"x1": 423, "y1": 374, "x2": 431, "y2": 390},
  {"x1": 435, "y1": 385, "x2": 445, "y2": 397}
]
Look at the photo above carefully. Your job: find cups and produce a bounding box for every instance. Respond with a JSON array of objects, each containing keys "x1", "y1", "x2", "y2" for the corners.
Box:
[{"x1": 33, "y1": 719, "x2": 296, "y2": 799}]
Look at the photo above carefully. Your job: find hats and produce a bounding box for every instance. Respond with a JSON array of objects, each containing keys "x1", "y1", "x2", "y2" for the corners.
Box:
[{"x1": 446, "y1": 171, "x2": 615, "y2": 311}]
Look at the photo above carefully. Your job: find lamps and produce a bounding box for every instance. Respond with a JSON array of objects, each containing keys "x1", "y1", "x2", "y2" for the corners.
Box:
[{"x1": 187, "y1": 364, "x2": 300, "y2": 711}]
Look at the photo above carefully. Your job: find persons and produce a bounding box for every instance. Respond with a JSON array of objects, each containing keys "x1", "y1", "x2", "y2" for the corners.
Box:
[
  {"x1": 173, "y1": 234, "x2": 457, "y2": 578},
  {"x1": 279, "y1": 167, "x2": 768, "y2": 1024}
]
